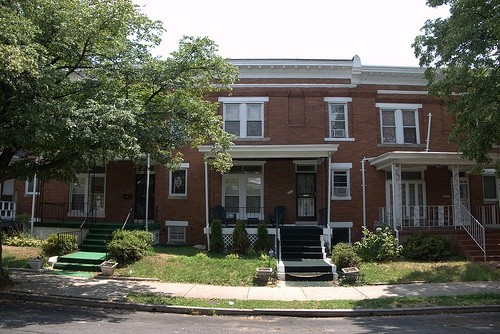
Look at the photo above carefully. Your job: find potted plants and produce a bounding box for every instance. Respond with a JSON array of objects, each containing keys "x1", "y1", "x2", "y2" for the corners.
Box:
[
  {"x1": 28, "y1": 255, "x2": 44, "y2": 269},
  {"x1": 255, "y1": 266, "x2": 273, "y2": 279},
  {"x1": 100, "y1": 260, "x2": 119, "y2": 275},
  {"x1": 342, "y1": 266, "x2": 360, "y2": 281}
]
